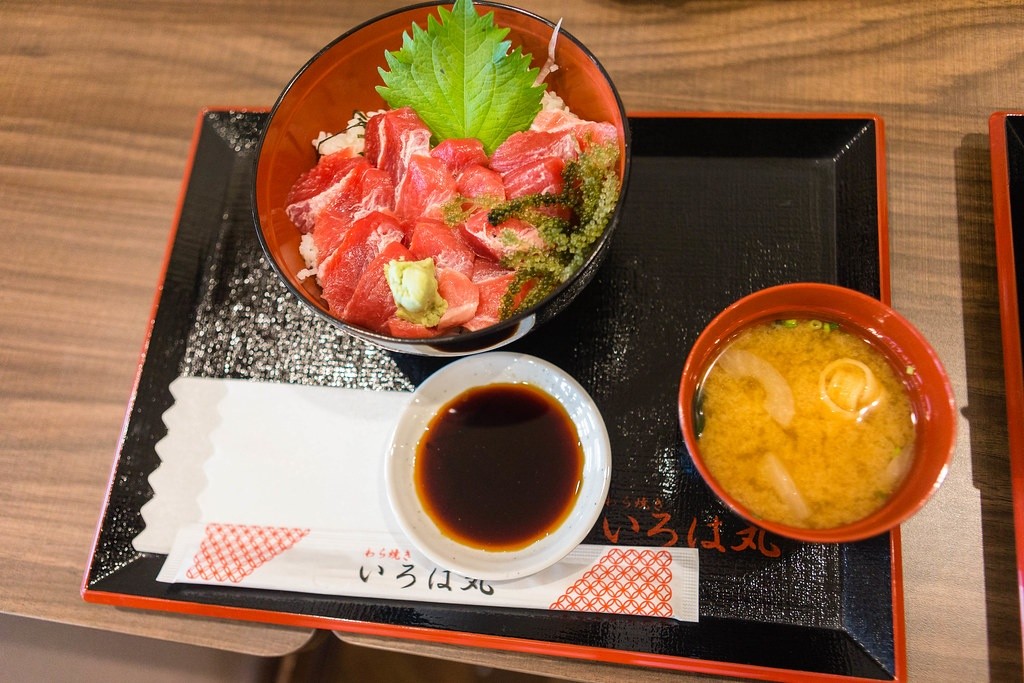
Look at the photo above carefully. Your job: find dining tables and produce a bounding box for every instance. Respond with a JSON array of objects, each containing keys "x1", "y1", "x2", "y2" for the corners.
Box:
[
  {"x1": 0, "y1": 0, "x2": 429, "y2": 661},
  {"x1": 328, "y1": 0, "x2": 1024, "y2": 683}
]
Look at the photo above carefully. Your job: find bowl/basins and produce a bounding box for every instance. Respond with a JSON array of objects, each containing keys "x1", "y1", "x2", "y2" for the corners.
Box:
[
  {"x1": 678, "y1": 281, "x2": 959, "y2": 543},
  {"x1": 249, "y1": 0, "x2": 632, "y2": 358},
  {"x1": 383, "y1": 351, "x2": 612, "y2": 581}
]
